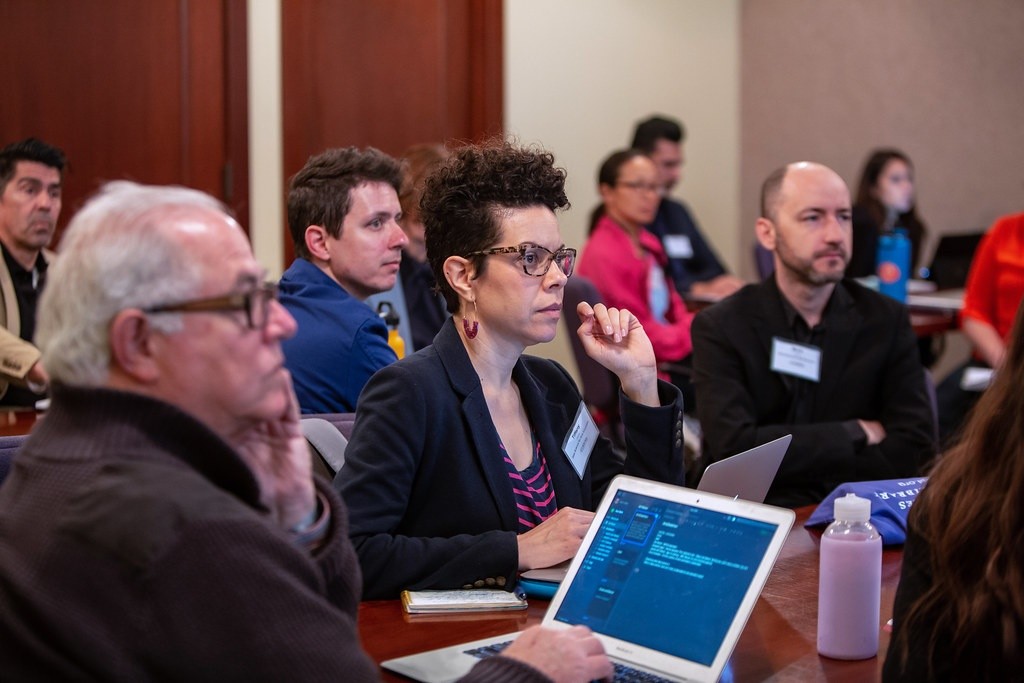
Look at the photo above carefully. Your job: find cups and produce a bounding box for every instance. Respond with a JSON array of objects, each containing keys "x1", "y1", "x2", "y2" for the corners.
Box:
[{"x1": 876, "y1": 235, "x2": 909, "y2": 302}]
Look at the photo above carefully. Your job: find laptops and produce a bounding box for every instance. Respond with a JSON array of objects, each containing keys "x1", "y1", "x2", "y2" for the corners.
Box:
[
  {"x1": 522, "y1": 434, "x2": 794, "y2": 583},
  {"x1": 380, "y1": 473, "x2": 796, "y2": 683},
  {"x1": 923, "y1": 232, "x2": 985, "y2": 291}
]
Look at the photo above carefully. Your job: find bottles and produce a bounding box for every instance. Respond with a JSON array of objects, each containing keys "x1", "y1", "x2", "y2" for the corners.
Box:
[
  {"x1": 814, "y1": 497, "x2": 881, "y2": 659},
  {"x1": 376, "y1": 300, "x2": 404, "y2": 362}
]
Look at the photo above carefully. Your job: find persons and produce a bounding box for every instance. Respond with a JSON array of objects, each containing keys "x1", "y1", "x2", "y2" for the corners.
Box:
[
  {"x1": 580, "y1": 114, "x2": 737, "y2": 413},
  {"x1": 0, "y1": 137, "x2": 73, "y2": 408},
  {"x1": 0, "y1": 181, "x2": 613, "y2": 682},
  {"x1": 878, "y1": 296, "x2": 1024, "y2": 682},
  {"x1": 271, "y1": 147, "x2": 410, "y2": 414},
  {"x1": 690, "y1": 161, "x2": 936, "y2": 516},
  {"x1": 844, "y1": 147, "x2": 927, "y2": 278},
  {"x1": 934, "y1": 210, "x2": 1024, "y2": 449},
  {"x1": 332, "y1": 146, "x2": 683, "y2": 594}
]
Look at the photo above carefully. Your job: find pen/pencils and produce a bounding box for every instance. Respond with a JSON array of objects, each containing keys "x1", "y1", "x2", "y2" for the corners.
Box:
[{"x1": 514, "y1": 584, "x2": 526, "y2": 601}]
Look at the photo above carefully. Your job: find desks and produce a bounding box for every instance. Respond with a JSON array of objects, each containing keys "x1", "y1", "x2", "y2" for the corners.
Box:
[
  {"x1": 355, "y1": 504, "x2": 930, "y2": 682},
  {"x1": 910, "y1": 287, "x2": 970, "y2": 335}
]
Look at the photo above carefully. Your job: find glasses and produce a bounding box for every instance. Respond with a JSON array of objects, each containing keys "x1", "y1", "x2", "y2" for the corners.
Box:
[
  {"x1": 615, "y1": 179, "x2": 664, "y2": 194},
  {"x1": 140, "y1": 282, "x2": 278, "y2": 330},
  {"x1": 463, "y1": 244, "x2": 576, "y2": 278}
]
